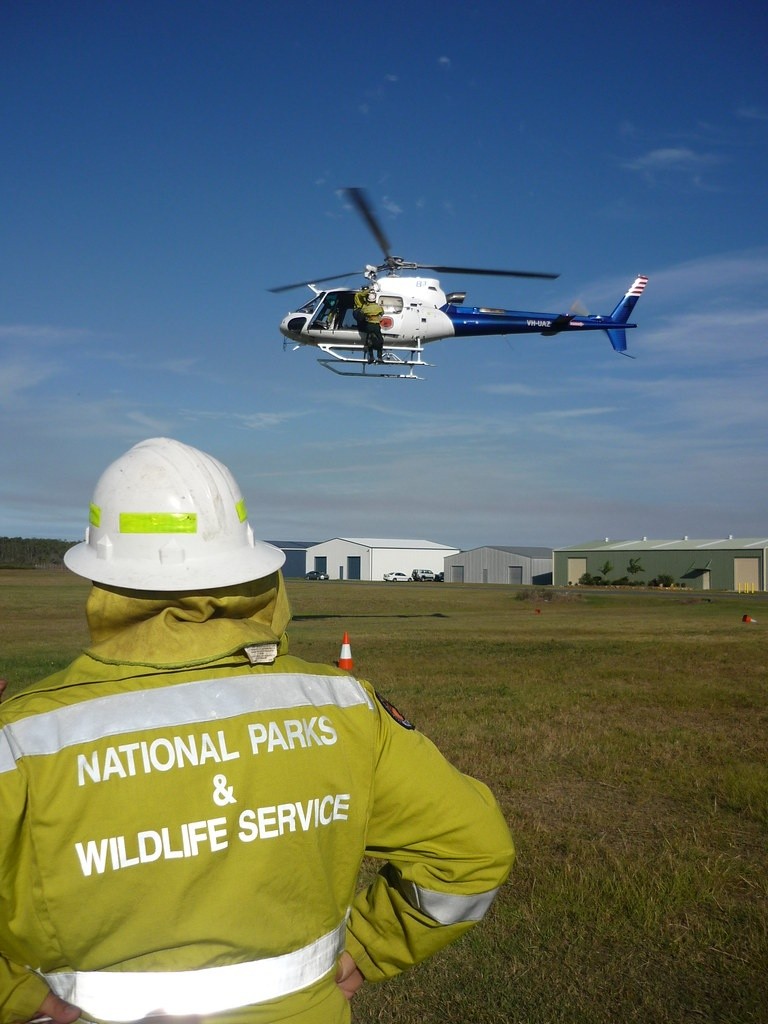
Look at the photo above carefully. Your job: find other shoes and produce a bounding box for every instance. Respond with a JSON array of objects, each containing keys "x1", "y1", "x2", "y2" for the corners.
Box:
[
  {"x1": 378, "y1": 358, "x2": 384, "y2": 363},
  {"x1": 359, "y1": 328, "x2": 363, "y2": 332},
  {"x1": 368, "y1": 357, "x2": 374, "y2": 364}
]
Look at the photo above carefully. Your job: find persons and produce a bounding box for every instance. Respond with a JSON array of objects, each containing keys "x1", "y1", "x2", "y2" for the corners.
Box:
[
  {"x1": 1, "y1": 437, "x2": 515, "y2": 1024},
  {"x1": 356, "y1": 292, "x2": 384, "y2": 364}
]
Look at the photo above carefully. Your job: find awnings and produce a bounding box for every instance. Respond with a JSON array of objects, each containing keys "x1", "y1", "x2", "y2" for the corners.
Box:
[{"x1": 691, "y1": 560, "x2": 712, "y2": 571}]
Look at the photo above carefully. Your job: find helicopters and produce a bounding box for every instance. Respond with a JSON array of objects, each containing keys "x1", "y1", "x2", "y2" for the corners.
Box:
[{"x1": 266, "y1": 188, "x2": 649, "y2": 380}]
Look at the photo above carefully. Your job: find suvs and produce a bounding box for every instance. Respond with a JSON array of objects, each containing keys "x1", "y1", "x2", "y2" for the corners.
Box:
[{"x1": 412, "y1": 569, "x2": 436, "y2": 582}]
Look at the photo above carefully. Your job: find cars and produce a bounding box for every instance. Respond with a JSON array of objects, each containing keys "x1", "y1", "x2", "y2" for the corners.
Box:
[
  {"x1": 435, "y1": 571, "x2": 444, "y2": 582},
  {"x1": 383, "y1": 572, "x2": 415, "y2": 583},
  {"x1": 304, "y1": 571, "x2": 329, "y2": 581}
]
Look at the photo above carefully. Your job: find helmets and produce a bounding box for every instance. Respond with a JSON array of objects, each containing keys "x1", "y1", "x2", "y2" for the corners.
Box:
[
  {"x1": 63, "y1": 437, "x2": 286, "y2": 592},
  {"x1": 367, "y1": 294, "x2": 376, "y2": 301},
  {"x1": 362, "y1": 286, "x2": 369, "y2": 290}
]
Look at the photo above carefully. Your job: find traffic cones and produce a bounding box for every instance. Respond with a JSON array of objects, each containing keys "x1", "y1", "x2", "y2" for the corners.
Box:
[
  {"x1": 338, "y1": 631, "x2": 353, "y2": 670},
  {"x1": 742, "y1": 615, "x2": 758, "y2": 623},
  {"x1": 534, "y1": 609, "x2": 541, "y2": 615}
]
